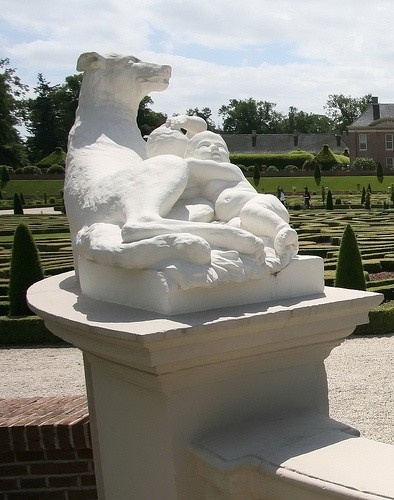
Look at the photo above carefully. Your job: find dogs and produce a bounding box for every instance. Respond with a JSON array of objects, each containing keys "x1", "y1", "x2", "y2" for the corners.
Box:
[{"x1": 62, "y1": 50, "x2": 266, "y2": 270}]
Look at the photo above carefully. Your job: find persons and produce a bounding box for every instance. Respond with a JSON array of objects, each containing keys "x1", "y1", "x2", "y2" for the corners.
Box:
[
  {"x1": 280, "y1": 190, "x2": 285, "y2": 204},
  {"x1": 145, "y1": 114, "x2": 208, "y2": 198},
  {"x1": 365, "y1": 189, "x2": 371, "y2": 209},
  {"x1": 184, "y1": 131, "x2": 299, "y2": 257},
  {"x1": 303, "y1": 187, "x2": 310, "y2": 206}
]
